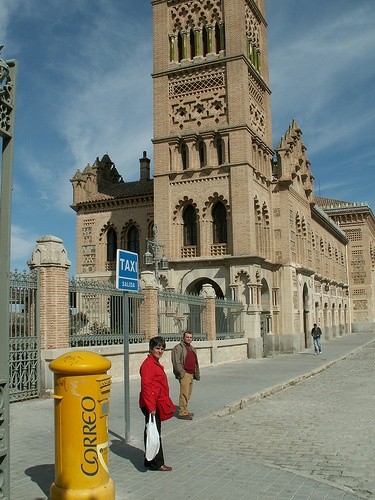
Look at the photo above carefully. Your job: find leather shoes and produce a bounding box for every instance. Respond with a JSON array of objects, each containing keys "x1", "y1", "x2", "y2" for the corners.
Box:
[
  {"x1": 158, "y1": 464, "x2": 173, "y2": 471},
  {"x1": 179, "y1": 415, "x2": 193, "y2": 420},
  {"x1": 188, "y1": 413, "x2": 194, "y2": 416}
]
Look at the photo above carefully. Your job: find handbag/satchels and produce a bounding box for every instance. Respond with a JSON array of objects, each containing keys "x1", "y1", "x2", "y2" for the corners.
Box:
[{"x1": 156, "y1": 401, "x2": 176, "y2": 422}]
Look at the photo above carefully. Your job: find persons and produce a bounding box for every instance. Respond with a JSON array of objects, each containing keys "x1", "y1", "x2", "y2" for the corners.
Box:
[
  {"x1": 139, "y1": 336, "x2": 175, "y2": 471},
  {"x1": 171, "y1": 331, "x2": 200, "y2": 420},
  {"x1": 311, "y1": 324, "x2": 322, "y2": 354}
]
